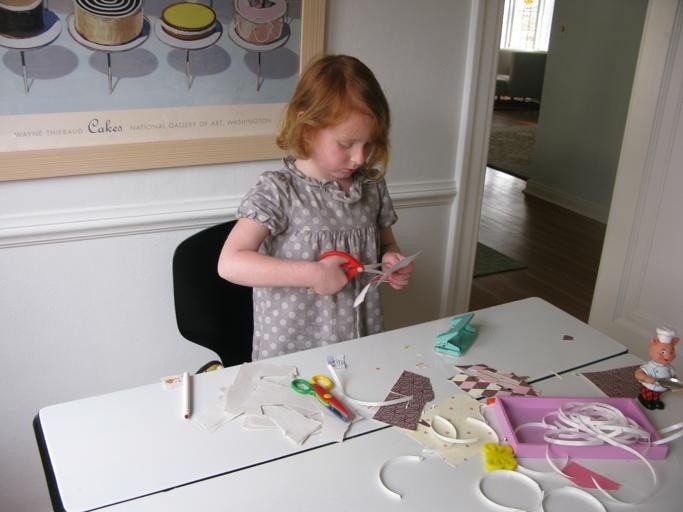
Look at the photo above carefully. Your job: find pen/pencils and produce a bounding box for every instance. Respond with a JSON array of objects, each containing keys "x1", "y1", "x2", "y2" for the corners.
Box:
[{"x1": 182, "y1": 371, "x2": 191, "y2": 419}]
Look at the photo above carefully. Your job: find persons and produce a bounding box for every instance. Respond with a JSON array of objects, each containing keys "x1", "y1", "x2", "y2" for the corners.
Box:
[{"x1": 217, "y1": 52, "x2": 415, "y2": 364}]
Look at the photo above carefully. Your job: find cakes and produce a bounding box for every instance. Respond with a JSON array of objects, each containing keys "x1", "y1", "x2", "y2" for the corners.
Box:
[
  {"x1": 161, "y1": 2, "x2": 218, "y2": 40},
  {"x1": 234, "y1": 0, "x2": 288, "y2": 45},
  {"x1": 74, "y1": 0, "x2": 144, "y2": 45},
  {"x1": 0, "y1": 1, "x2": 43, "y2": 36}
]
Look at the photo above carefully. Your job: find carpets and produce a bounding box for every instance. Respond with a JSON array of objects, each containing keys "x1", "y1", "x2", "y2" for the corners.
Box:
[{"x1": 469, "y1": 239, "x2": 529, "y2": 284}]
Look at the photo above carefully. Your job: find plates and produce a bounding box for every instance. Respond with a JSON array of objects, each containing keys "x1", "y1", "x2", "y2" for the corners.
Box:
[
  {"x1": 226, "y1": 19, "x2": 291, "y2": 53},
  {"x1": 153, "y1": 18, "x2": 224, "y2": 51},
  {"x1": 0, "y1": 9, "x2": 63, "y2": 49},
  {"x1": 66, "y1": 11, "x2": 152, "y2": 53}
]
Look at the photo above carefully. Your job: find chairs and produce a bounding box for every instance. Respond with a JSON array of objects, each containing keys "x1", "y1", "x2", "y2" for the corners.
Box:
[{"x1": 170, "y1": 217, "x2": 272, "y2": 375}]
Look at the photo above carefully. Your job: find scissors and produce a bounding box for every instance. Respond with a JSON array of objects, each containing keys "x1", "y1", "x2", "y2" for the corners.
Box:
[
  {"x1": 319, "y1": 250, "x2": 390, "y2": 284},
  {"x1": 291, "y1": 375, "x2": 350, "y2": 422}
]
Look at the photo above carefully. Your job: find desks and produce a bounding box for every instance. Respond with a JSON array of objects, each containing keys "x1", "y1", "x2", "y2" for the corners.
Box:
[{"x1": 30, "y1": 292, "x2": 681, "y2": 512}]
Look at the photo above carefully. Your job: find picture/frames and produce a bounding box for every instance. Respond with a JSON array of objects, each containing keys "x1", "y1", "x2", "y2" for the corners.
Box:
[{"x1": 0, "y1": 0, "x2": 328, "y2": 185}]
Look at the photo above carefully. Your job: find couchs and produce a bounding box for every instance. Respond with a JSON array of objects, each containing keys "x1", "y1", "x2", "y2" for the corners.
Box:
[{"x1": 490, "y1": 49, "x2": 548, "y2": 104}]
